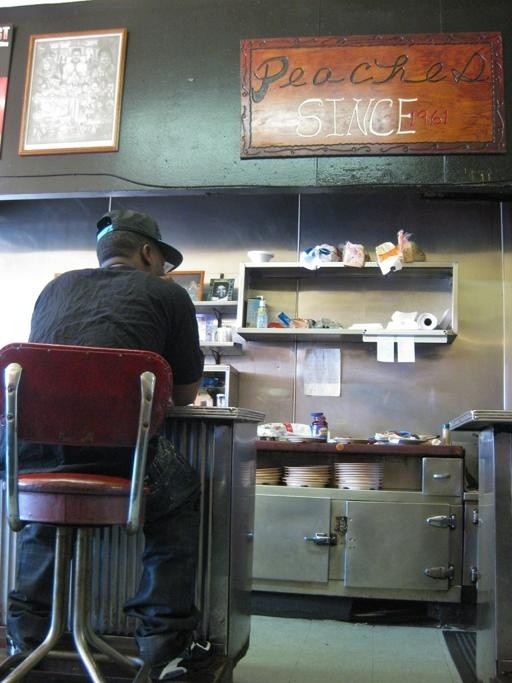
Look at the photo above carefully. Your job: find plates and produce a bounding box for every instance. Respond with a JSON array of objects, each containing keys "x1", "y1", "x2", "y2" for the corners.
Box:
[
  {"x1": 237, "y1": 460, "x2": 385, "y2": 490},
  {"x1": 282, "y1": 434, "x2": 326, "y2": 442},
  {"x1": 333, "y1": 437, "x2": 370, "y2": 444},
  {"x1": 374, "y1": 434, "x2": 428, "y2": 445}
]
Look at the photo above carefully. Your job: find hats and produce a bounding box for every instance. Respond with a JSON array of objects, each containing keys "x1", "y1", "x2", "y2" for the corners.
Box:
[{"x1": 95, "y1": 210, "x2": 182, "y2": 274}]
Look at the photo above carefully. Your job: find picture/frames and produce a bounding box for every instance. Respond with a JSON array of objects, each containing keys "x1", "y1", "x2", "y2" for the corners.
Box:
[
  {"x1": 161, "y1": 270, "x2": 205, "y2": 301},
  {"x1": 239, "y1": 30, "x2": 507, "y2": 160},
  {"x1": 17, "y1": 27, "x2": 127, "y2": 156}
]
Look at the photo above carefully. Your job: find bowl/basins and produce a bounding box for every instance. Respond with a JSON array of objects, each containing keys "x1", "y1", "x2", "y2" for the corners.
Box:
[{"x1": 247, "y1": 250, "x2": 275, "y2": 261}]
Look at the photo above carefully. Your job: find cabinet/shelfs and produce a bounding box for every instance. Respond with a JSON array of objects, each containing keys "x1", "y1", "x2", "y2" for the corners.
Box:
[{"x1": 192, "y1": 262, "x2": 460, "y2": 407}]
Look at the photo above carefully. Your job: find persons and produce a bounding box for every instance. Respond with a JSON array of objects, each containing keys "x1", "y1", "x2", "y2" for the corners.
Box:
[
  {"x1": 26, "y1": 39, "x2": 114, "y2": 144},
  {"x1": 211, "y1": 280, "x2": 228, "y2": 301},
  {"x1": 0, "y1": 207, "x2": 228, "y2": 680}
]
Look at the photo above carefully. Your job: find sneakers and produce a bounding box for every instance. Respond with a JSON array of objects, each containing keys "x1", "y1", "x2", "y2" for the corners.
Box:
[
  {"x1": 5, "y1": 637, "x2": 45, "y2": 669},
  {"x1": 149, "y1": 640, "x2": 227, "y2": 683}
]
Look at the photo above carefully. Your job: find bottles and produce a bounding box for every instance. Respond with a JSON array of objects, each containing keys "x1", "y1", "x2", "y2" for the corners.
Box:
[
  {"x1": 441, "y1": 422, "x2": 452, "y2": 446},
  {"x1": 195, "y1": 311, "x2": 208, "y2": 345}
]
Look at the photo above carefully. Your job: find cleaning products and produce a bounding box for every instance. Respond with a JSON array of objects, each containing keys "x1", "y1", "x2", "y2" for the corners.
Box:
[{"x1": 256, "y1": 295, "x2": 268, "y2": 328}]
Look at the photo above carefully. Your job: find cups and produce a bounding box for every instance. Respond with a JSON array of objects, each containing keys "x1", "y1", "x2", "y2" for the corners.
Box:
[{"x1": 211, "y1": 327, "x2": 233, "y2": 342}]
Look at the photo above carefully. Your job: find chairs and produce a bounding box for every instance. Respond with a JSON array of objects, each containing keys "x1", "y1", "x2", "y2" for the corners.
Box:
[{"x1": 0, "y1": 342, "x2": 174, "y2": 683}]
